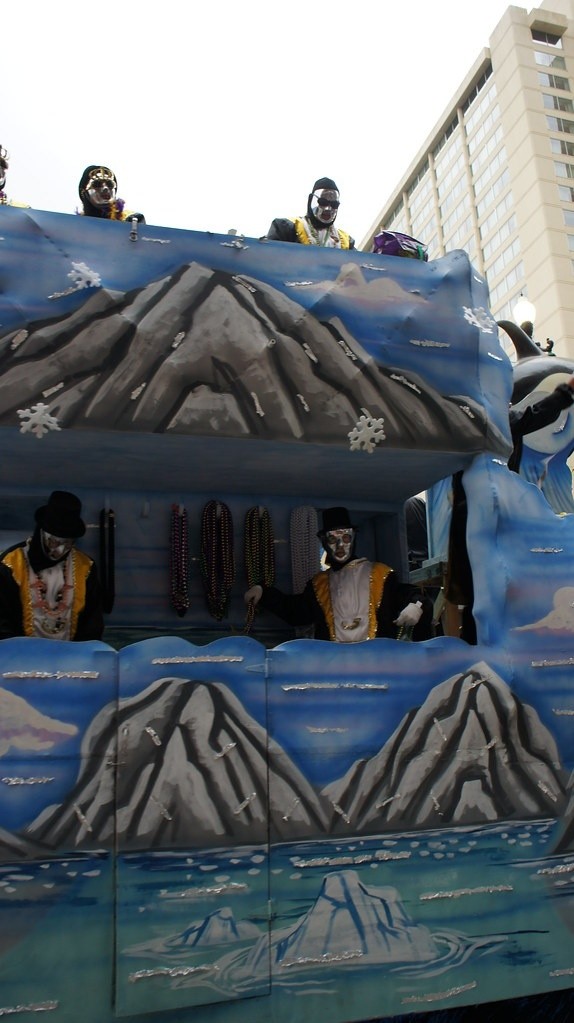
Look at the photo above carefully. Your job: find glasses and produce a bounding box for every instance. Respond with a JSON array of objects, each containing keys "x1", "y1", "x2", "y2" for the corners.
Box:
[
  {"x1": 313, "y1": 194, "x2": 340, "y2": 210},
  {"x1": 84, "y1": 179, "x2": 114, "y2": 192}
]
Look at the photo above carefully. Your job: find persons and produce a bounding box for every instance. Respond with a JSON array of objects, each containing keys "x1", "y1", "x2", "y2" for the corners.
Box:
[
  {"x1": 0, "y1": 490, "x2": 105, "y2": 642},
  {"x1": 75, "y1": 163, "x2": 145, "y2": 223},
  {"x1": 0, "y1": 144, "x2": 31, "y2": 210},
  {"x1": 267, "y1": 175, "x2": 359, "y2": 252},
  {"x1": 403, "y1": 370, "x2": 574, "y2": 646},
  {"x1": 244, "y1": 507, "x2": 433, "y2": 642}
]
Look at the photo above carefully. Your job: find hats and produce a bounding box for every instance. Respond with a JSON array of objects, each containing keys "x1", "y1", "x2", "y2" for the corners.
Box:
[
  {"x1": 35, "y1": 491, "x2": 87, "y2": 538},
  {"x1": 316, "y1": 506, "x2": 356, "y2": 534}
]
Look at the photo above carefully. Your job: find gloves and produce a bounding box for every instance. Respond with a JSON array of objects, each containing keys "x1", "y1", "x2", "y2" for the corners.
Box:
[
  {"x1": 393, "y1": 600, "x2": 425, "y2": 627},
  {"x1": 244, "y1": 585, "x2": 262, "y2": 606}
]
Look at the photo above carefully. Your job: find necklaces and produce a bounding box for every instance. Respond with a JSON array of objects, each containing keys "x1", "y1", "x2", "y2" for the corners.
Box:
[
  {"x1": 30, "y1": 558, "x2": 72, "y2": 634},
  {"x1": 168, "y1": 502, "x2": 322, "y2": 640},
  {"x1": 98, "y1": 508, "x2": 117, "y2": 614},
  {"x1": 397, "y1": 625, "x2": 414, "y2": 641}
]
